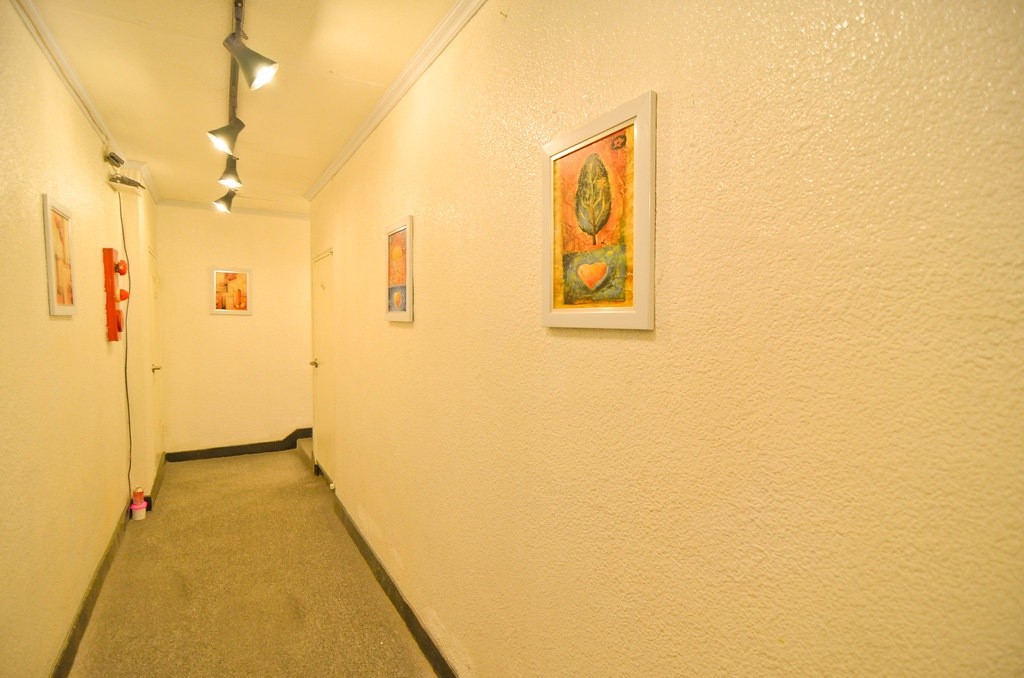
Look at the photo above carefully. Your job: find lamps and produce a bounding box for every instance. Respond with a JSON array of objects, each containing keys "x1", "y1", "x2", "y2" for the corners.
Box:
[
  {"x1": 108, "y1": 174, "x2": 146, "y2": 193},
  {"x1": 205, "y1": 1, "x2": 278, "y2": 212}
]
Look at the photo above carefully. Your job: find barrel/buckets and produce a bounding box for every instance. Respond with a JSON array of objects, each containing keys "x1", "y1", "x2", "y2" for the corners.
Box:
[{"x1": 131, "y1": 501, "x2": 148, "y2": 520}]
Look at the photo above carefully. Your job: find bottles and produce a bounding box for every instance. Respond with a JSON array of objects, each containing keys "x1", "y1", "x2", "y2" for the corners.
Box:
[{"x1": 132, "y1": 487, "x2": 144, "y2": 506}]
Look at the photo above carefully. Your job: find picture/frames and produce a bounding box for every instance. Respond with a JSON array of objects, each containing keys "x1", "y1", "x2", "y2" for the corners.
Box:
[
  {"x1": 42, "y1": 194, "x2": 78, "y2": 316},
  {"x1": 385, "y1": 214, "x2": 413, "y2": 324},
  {"x1": 536, "y1": 87, "x2": 658, "y2": 332},
  {"x1": 208, "y1": 266, "x2": 255, "y2": 316}
]
